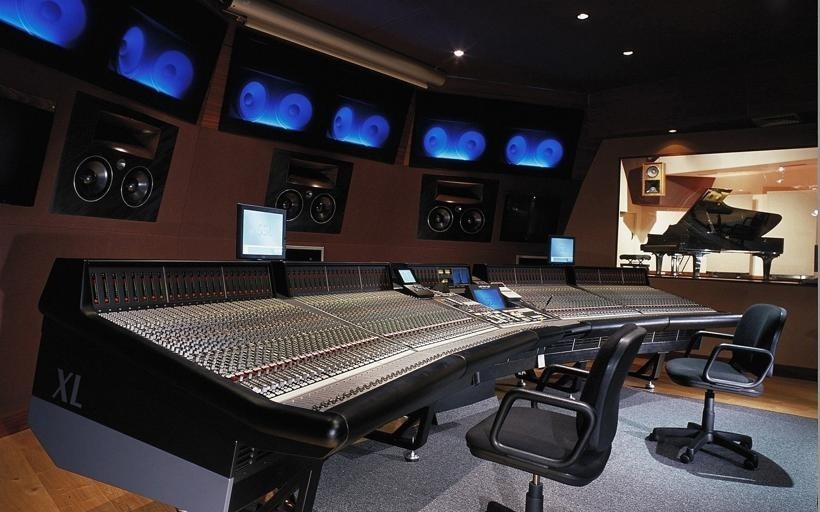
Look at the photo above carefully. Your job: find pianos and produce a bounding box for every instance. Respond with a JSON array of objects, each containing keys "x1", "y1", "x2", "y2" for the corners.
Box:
[{"x1": 641, "y1": 188, "x2": 784, "y2": 281}]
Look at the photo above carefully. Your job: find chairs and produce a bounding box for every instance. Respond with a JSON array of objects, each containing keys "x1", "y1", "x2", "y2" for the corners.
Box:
[
  {"x1": 465, "y1": 321, "x2": 648, "y2": 511},
  {"x1": 644, "y1": 301, "x2": 789, "y2": 471}
]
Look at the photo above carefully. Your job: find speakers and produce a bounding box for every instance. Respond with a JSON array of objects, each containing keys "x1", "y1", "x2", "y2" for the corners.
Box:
[
  {"x1": 0, "y1": 1, "x2": 87, "y2": 79},
  {"x1": 264, "y1": 148, "x2": 353, "y2": 234},
  {"x1": 416, "y1": 173, "x2": 495, "y2": 245},
  {"x1": 498, "y1": 126, "x2": 575, "y2": 179},
  {"x1": 90, "y1": 0, "x2": 214, "y2": 124},
  {"x1": 643, "y1": 162, "x2": 665, "y2": 198},
  {"x1": 50, "y1": 91, "x2": 181, "y2": 223},
  {"x1": 411, "y1": 115, "x2": 492, "y2": 170},
  {"x1": 218, "y1": 62, "x2": 320, "y2": 148},
  {"x1": 320, "y1": 95, "x2": 399, "y2": 164}
]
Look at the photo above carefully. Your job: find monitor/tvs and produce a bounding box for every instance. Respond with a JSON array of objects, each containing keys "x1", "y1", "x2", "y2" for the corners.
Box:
[
  {"x1": 234, "y1": 204, "x2": 286, "y2": 260},
  {"x1": 468, "y1": 282, "x2": 506, "y2": 309},
  {"x1": 547, "y1": 234, "x2": 575, "y2": 265}
]
